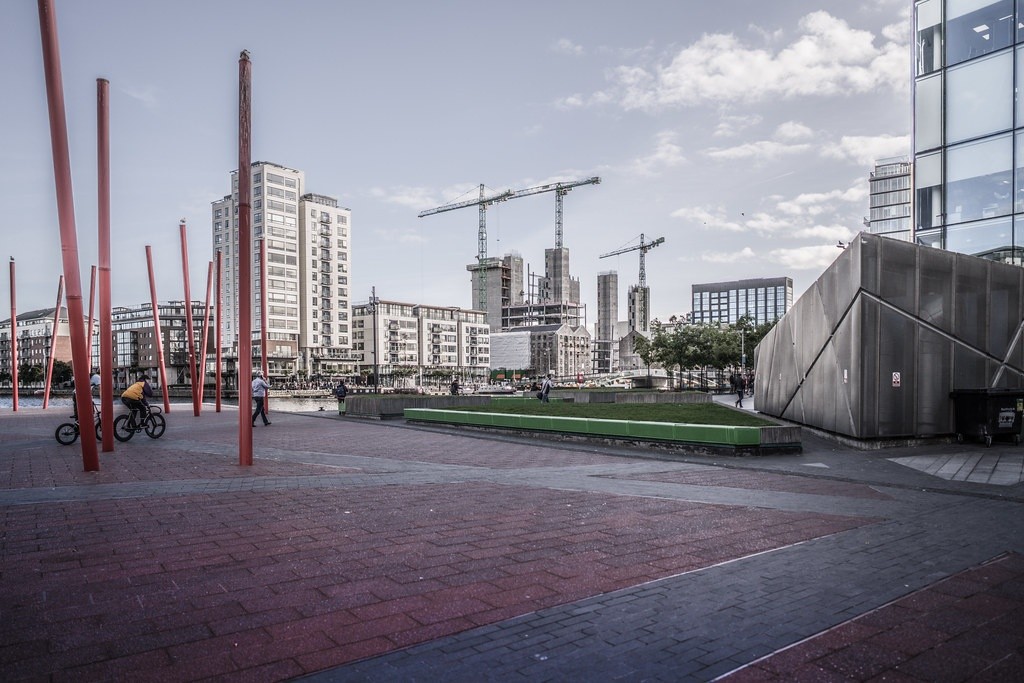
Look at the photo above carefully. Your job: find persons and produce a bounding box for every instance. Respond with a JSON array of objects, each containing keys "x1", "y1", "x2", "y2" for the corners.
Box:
[
  {"x1": 252, "y1": 371, "x2": 272, "y2": 427},
  {"x1": 337, "y1": 381, "x2": 348, "y2": 415},
  {"x1": 73, "y1": 368, "x2": 101, "y2": 425},
  {"x1": 451, "y1": 380, "x2": 462, "y2": 395},
  {"x1": 540, "y1": 374, "x2": 555, "y2": 404},
  {"x1": 531, "y1": 382, "x2": 540, "y2": 391},
  {"x1": 729, "y1": 372, "x2": 734, "y2": 395},
  {"x1": 735, "y1": 374, "x2": 743, "y2": 407},
  {"x1": 121, "y1": 375, "x2": 153, "y2": 428},
  {"x1": 745, "y1": 375, "x2": 753, "y2": 395}
]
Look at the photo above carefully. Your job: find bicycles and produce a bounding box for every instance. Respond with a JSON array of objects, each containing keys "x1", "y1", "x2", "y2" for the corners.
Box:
[
  {"x1": 113, "y1": 400, "x2": 166, "y2": 442},
  {"x1": 55, "y1": 402, "x2": 105, "y2": 445}
]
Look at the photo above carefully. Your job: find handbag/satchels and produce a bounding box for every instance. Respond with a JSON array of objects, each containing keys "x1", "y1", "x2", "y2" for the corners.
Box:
[{"x1": 535, "y1": 391, "x2": 543, "y2": 399}]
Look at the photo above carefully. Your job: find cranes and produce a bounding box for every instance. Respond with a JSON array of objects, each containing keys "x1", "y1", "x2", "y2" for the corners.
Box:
[
  {"x1": 418, "y1": 183, "x2": 515, "y2": 323},
  {"x1": 599, "y1": 232, "x2": 665, "y2": 331},
  {"x1": 505, "y1": 176, "x2": 602, "y2": 249}
]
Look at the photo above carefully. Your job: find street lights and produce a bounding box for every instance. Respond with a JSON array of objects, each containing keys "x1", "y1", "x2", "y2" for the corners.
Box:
[{"x1": 368, "y1": 285, "x2": 381, "y2": 393}]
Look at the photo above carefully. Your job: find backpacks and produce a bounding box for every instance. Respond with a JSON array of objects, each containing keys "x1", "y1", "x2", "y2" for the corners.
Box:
[
  {"x1": 336, "y1": 385, "x2": 345, "y2": 397},
  {"x1": 449, "y1": 385, "x2": 456, "y2": 395}
]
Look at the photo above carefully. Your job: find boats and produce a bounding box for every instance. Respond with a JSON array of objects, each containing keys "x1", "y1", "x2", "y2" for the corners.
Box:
[{"x1": 479, "y1": 382, "x2": 517, "y2": 394}]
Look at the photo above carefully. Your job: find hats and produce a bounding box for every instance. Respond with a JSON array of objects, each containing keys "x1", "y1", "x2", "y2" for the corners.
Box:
[
  {"x1": 97, "y1": 368, "x2": 100, "y2": 372},
  {"x1": 256, "y1": 371, "x2": 263, "y2": 376},
  {"x1": 139, "y1": 374, "x2": 150, "y2": 379}
]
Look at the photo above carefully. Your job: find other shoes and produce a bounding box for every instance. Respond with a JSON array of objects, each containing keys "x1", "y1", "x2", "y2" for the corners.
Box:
[
  {"x1": 265, "y1": 422, "x2": 271, "y2": 425},
  {"x1": 252, "y1": 425, "x2": 256, "y2": 427}
]
